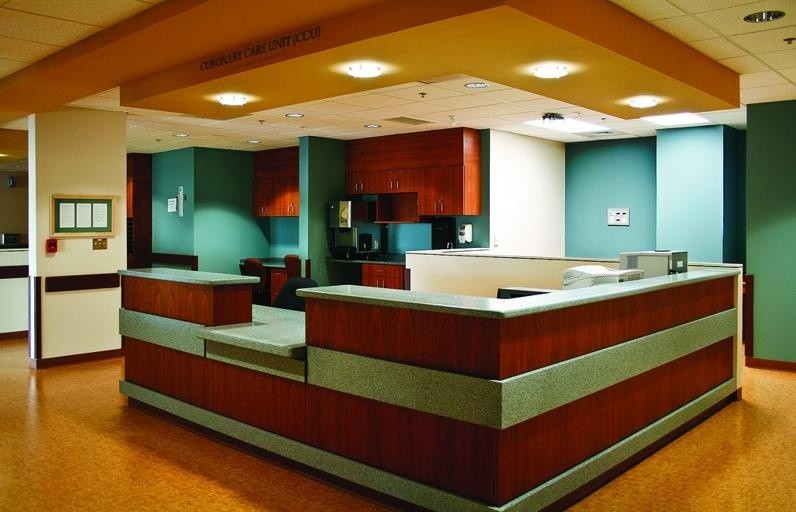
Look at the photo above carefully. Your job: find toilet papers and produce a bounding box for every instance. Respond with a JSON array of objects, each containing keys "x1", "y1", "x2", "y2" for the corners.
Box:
[{"x1": 457, "y1": 224, "x2": 473, "y2": 244}]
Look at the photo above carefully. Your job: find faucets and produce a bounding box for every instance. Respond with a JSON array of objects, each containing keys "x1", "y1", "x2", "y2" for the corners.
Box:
[{"x1": 447, "y1": 241, "x2": 454, "y2": 249}]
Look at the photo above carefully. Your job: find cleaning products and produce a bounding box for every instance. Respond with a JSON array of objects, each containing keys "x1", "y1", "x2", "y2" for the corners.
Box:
[{"x1": 374, "y1": 238, "x2": 379, "y2": 250}]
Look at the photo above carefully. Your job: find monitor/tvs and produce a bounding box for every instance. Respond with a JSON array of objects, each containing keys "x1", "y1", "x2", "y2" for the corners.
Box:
[{"x1": 561, "y1": 265, "x2": 645, "y2": 290}]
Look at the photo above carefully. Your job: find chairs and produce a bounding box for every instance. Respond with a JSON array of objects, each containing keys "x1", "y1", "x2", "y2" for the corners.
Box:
[
  {"x1": 272, "y1": 276, "x2": 320, "y2": 311},
  {"x1": 241, "y1": 258, "x2": 267, "y2": 296},
  {"x1": 284, "y1": 254, "x2": 300, "y2": 282}
]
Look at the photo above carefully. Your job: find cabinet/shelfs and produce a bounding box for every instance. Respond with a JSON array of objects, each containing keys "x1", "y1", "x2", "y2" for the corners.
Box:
[
  {"x1": 416, "y1": 127, "x2": 482, "y2": 216},
  {"x1": 275, "y1": 148, "x2": 298, "y2": 216},
  {"x1": 370, "y1": 135, "x2": 431, "y2": 224},
  {"x1": 253, "y1": 149, "x2": 275, "y2": 216},
  {"x1": 243, "y1": 257, "x2": 301, "y2": 302},
  {"x1": 345, "y1": 137, "x2": 370, "y2": 195},
  {"x1": 361, "y1": 264, "x2": 410, "y2": 291}
]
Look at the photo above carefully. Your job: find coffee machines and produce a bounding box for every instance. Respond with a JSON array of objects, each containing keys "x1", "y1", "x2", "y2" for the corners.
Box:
[{"x1": 329, "y1": 201, "x2": 383, "y2": 259}]
